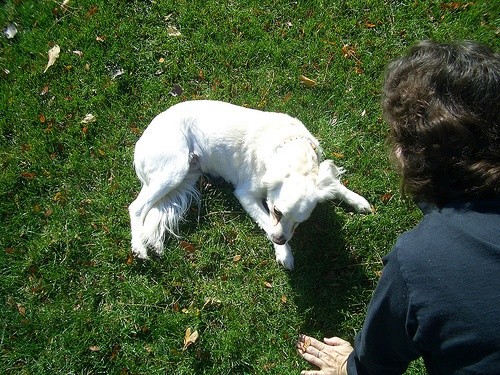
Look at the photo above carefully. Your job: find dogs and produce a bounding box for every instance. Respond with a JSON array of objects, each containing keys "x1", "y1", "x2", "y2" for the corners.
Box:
[{"x1": 126, "y1": 100, "x2": 373, "y2": 272}]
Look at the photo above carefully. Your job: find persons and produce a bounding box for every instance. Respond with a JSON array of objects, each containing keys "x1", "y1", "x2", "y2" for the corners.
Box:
[{"x1": 296, "y1": 39, "x2": 500, "y2": 375}]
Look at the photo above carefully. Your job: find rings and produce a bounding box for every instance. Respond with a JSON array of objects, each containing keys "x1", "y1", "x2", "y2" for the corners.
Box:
[{"x1": 316, "y1": 351, "x2": 320, "y2": 358}]
